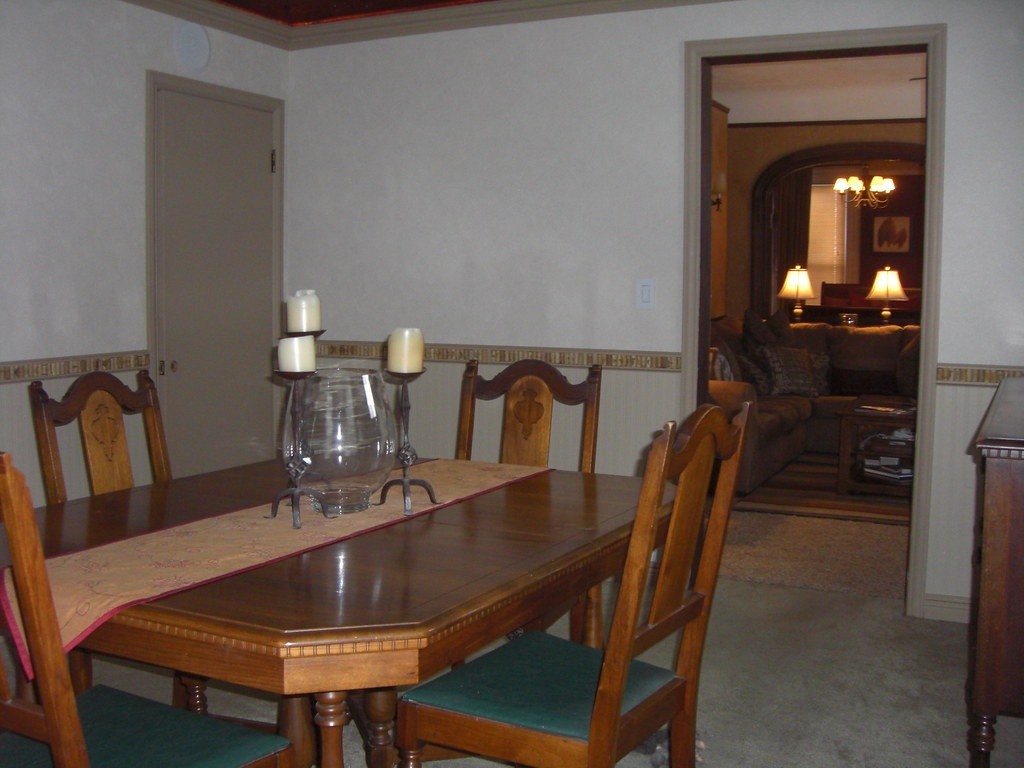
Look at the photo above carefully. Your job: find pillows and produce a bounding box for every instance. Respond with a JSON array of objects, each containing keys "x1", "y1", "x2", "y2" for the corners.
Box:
[{"x1": 709, "y1": 310, "x2": 831, "y2": 397}]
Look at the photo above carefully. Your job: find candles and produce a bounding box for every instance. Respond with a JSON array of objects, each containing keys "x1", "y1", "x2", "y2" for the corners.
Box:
[
  {"x1": 285, "y1": 290, "x2": 321, "y2": 332},
  {"x1": 278, "y1": 335, "x2": 316, "y2": 371},
  {"x1": 387, "y1": 328, "x2": 423, "y2": 373}
]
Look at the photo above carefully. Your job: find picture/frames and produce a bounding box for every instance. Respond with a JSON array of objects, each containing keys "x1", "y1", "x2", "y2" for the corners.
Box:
[{"x1": 871, "y1": 215, "x2": 911, "y2": 255}]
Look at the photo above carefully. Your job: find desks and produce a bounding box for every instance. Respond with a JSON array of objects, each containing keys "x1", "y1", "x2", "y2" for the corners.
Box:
[
  {"x1": 837, "y1": 396, "x2": 916, "y2": 495},
  {"x1": 969, "y1": 375, "x2": 1024, "y2": 768},
  {"x1": 0, "y1": 453, "x2": 680, "y2": 768}
]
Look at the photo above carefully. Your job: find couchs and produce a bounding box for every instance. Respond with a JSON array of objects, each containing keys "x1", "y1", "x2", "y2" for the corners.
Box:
[
  {"x1": 708, "y1": 314, "x2": 921, "y2": 494},
  {"x1": 820, "y1": 280, "x2": 921, "y2": 311}
]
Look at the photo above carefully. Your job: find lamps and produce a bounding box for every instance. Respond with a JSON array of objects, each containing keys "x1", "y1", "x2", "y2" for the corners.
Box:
[
  {"x1": 833, "y1": 168, "x2": 896, "y2": 210},
  {"x1": 778, "y1": 265, "x2": 816, "y2": 321},
  {"x1": 712, "y1": 171, "x2": 726, "y2": 211},
  {"x1": 864, "y1": 266, "x2": 909, "y2": 324}
]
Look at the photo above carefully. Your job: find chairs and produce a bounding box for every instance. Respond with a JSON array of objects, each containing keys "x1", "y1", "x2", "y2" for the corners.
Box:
[
  {"x1": 0, "y1": 450, "x2": 294, "y2": 767},
  {"x1": 395, "y1": 400, "x2": 753, "y2": 768},
  {"x1": 450, "y1": 359, "x2": 602, "y2": 669},
  {"x1": 29, "y1": 369, "x2": 190, "y2": 709}
]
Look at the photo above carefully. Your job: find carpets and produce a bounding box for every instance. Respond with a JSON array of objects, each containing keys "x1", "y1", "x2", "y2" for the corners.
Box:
[{"x1": 729, "y1": 452, "x2": 912, "y2": 526}]
